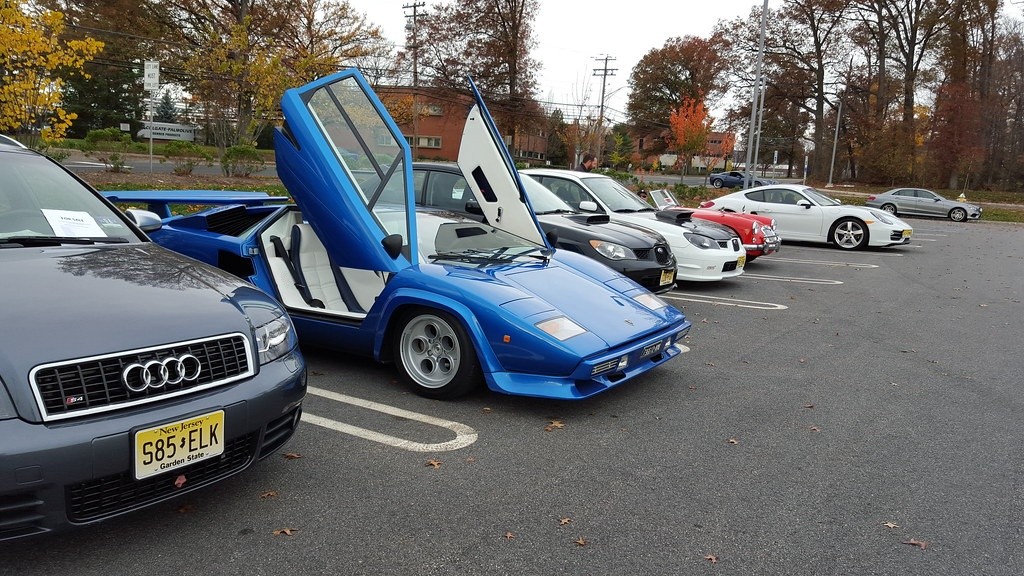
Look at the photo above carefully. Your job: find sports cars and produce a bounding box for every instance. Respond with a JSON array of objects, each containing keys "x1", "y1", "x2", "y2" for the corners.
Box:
[{"x1": 97, "y1": 64, "x2": 693, "y2": 407}]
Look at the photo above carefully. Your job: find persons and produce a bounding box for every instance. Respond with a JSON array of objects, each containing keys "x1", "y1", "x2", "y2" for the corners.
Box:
[
  {"x1": 636, "y1": 189, "x2": 648, "y2": 202},
  {"x1": 567, "y1": 154, "x2": 598, "y2": 203}
]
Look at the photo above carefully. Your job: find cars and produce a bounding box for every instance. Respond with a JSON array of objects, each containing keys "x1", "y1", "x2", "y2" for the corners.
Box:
[
  {"x1": 518, "y1": 168, "x2": 746, "y2": 283},
  {"x1": 865, "y1": 186, "x2": 984, "y2": 223},
  {"x1": 698, "y1": 183, "x2": 914, "y2": 251},
  {"x1": 0, "y1": 131, "x2": 309, "y2": 553},
  {"x1": 356, "y1": 160, "x2": 680, "y2": 296},
  {"x1": 647, "y1": 188, "x2": 782, "y2": 266},
  {"x1": 710, "y1": 170, "x2": 778, "y2": 190}
]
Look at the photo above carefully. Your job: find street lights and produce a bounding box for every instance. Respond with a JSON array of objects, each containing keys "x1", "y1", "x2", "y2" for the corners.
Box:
[{"x1": 825, "y1": 101, "x2": 843, "y2": 189}]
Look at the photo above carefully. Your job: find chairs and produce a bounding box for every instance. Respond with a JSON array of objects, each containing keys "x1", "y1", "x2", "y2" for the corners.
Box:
[
  {"x1": 292, "y1": 224, "x2": 366, "y2": 317},
  {"x1": 340, "y1": 267, "x2": 389, "y2": 313}
]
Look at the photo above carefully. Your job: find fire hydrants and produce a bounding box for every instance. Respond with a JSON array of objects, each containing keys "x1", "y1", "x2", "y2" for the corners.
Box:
[{"x1": 957, "y1": 193, "x2": 967, "y2": 203}]
